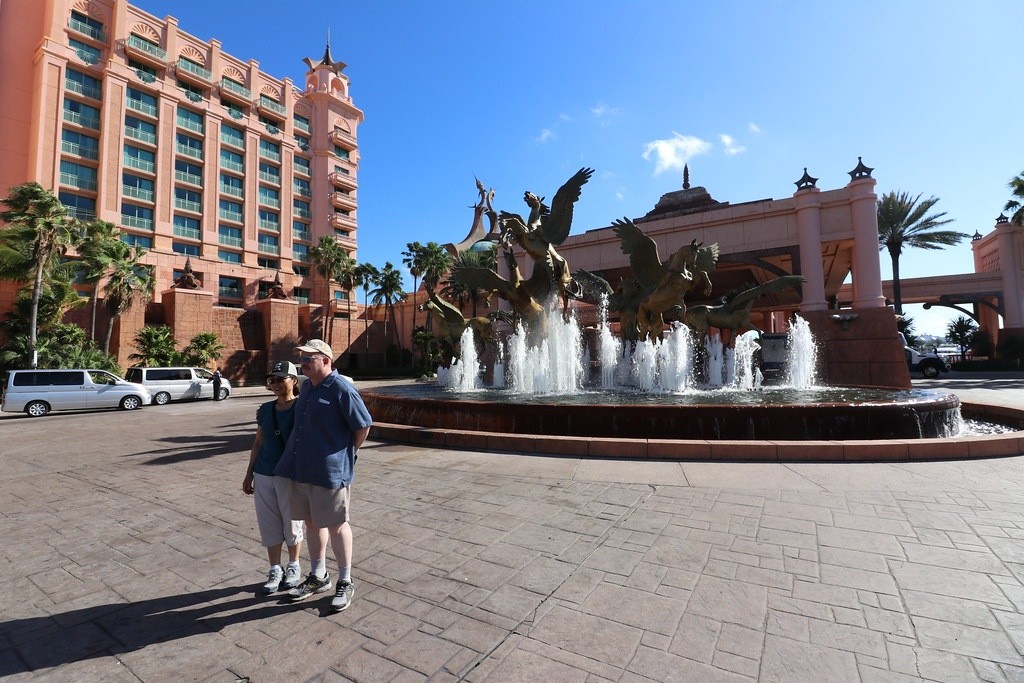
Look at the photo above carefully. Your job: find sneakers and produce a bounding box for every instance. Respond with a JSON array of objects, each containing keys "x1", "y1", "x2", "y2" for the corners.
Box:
[
  {"x1": 262, "y1": 566, "x2": 286, "y2": 595},
  {"x1": 329, "y1": 578, "x2": 354, "y2": 612},
  {"x1": 287, "y1": 571, "x2": 332, "y2": 602},
  {"x1": 283, "y1": 564, "x2": 301, "y2": 590}
]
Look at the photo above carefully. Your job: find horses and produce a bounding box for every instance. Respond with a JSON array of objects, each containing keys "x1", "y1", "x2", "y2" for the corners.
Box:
[{"x1": 417, "y1": 166, "x2": 808, "y2": 370}]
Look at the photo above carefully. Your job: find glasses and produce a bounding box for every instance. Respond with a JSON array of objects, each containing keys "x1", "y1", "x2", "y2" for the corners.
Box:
[
  {"x1": 267, "y1": 377, "x2": 295, "y2": 384},
  {"x1": 298, "y1": 355, "x2": 323, "y2": 362}
]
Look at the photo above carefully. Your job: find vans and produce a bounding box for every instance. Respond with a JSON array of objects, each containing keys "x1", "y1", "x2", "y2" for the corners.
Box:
[
  {"x1": 1, "y1": 369, "x2": 150, "y2": 418},
  {"x1": 124, "y1": 366, "x2": 231, "y2": 405}
]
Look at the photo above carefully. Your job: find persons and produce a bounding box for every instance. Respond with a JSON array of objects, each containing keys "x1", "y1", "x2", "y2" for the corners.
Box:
[
  {"x1": 291, "y1": 339, "x2": 374, "y2": 612},
  {"x1": 241, "y1": 362, "x2": 304, "y2": 594},
  {"x1": 212, "y1": 367, "x2": 222, "y2": 401}
]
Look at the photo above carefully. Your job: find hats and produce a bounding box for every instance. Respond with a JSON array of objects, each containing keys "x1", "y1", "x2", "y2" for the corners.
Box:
[
  {"x1": 292, "y1": 338, "x2": 333, "y2": 359},
  {"x1": 267, "y1": 361, "x2": 297, "y2": 379}
]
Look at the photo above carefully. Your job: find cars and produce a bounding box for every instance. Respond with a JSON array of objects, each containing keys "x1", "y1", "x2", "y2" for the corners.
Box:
[
  {"x1": 933, "y1": 343, "x2": 967, "y2": 360},
  {"x1": 904, "y1": 345, "x2": 950, "y2": 377},
  {"x1": 266, "y1": 364, "x2": 354, "y2": 395}
]
